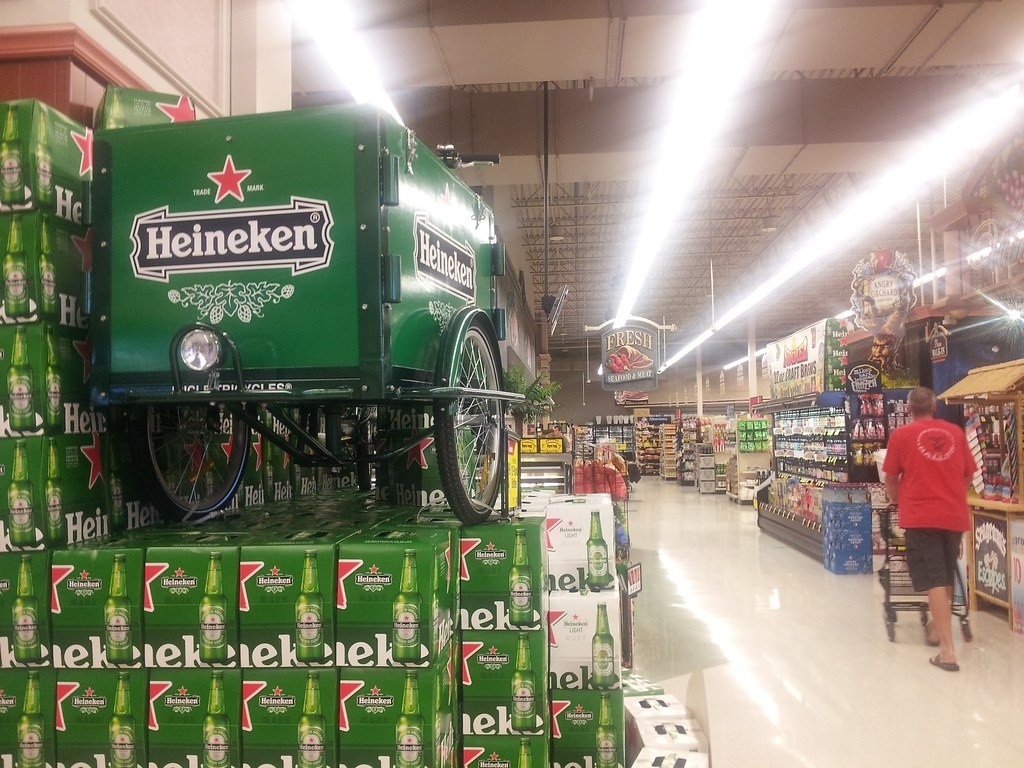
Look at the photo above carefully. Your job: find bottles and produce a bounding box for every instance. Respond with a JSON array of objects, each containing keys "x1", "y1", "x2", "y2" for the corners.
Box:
[{"x1": 0, "y1": 100, "x2": 625, "y2": 768}]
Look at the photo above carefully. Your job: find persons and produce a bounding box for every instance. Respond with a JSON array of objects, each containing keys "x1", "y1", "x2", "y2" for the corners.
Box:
[
  {"x1": 626, "y1": 454, "x2": 641, "y2": 492},
  {"x1": 882, "y1": 387, "x2": 979, "y2": 672},
  {"x1": 553, "y1": 425, "x2": 570, "y2": 453}
]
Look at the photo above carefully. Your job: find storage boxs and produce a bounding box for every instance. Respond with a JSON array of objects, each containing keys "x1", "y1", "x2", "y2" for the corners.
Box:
[{"x1": 0, "y1": 84, "x2": 712, "y2": 768}]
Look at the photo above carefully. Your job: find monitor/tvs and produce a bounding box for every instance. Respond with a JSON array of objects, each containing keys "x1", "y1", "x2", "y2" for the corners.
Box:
[{"x1": 548, "y1": 284, "x2": 567, "y2": 335}]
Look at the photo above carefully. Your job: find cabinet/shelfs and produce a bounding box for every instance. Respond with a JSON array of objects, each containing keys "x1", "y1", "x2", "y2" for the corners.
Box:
[{"x1": 573, "y1": 420, "x2": 771, "y2": 505}]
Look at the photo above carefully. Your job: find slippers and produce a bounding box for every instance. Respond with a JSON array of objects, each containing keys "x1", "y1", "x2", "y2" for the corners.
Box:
[
  {"x1": 929, "y1": 655, "x2": 959, "y2": 671},
  {"x1": 925, "y1": 620, "x2": 940, "y2": 648}
]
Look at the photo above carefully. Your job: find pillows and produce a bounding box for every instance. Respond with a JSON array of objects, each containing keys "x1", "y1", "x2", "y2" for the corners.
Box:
[{"x1": 614, "y1": 515, "x2": 629, "y2": 545}]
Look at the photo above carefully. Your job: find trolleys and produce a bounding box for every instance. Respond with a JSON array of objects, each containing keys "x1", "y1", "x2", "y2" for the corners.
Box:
[
  {"x1": 877, "y1": 504, "x2": 975, "y2": 643},
  {"x1": 94, "y1": 102, "x2": 529, "y2": 527}
]
